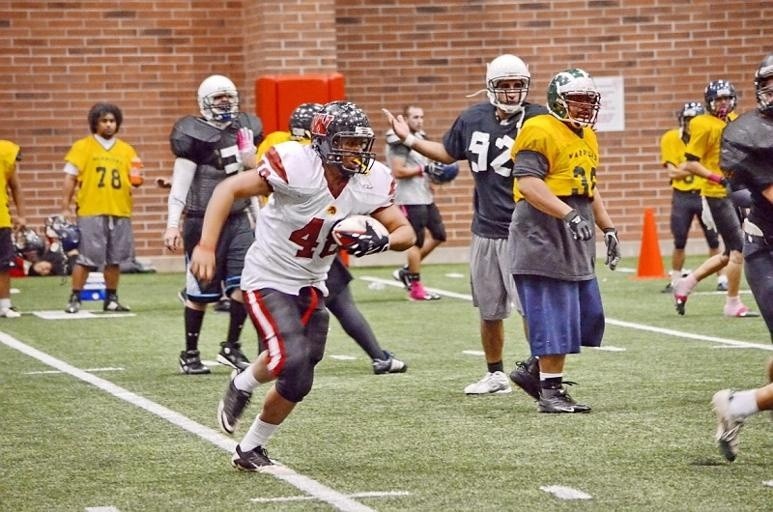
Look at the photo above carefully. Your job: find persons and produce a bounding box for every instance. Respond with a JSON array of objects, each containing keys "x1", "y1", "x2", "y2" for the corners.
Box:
[
  {"x1": 27, "y1": 224, "x2": 158, "y2": 280},
  {"x1": 190, "y1": 100, "x2": 417, "y2": 476},
  {"x1": 660, "y1": 103, "x2": 730, "y2": 292},
  {"x1": 509, "y1": 67, "x2": 621, "y2": 414},
  {"x1": 236, "y1": 103, "x2": 410, "y2": 376},
  {"x1": 163, "y1": 74, "x2": 265, "y2": 376},
  {"x1": 385, "y1": 105, "x2": 446, "y2": 302},
  {"x1": 0, "y1": 139, "x2": 27, "y2": 318},
  {"x1": 61, "y1": 102, "x2": 145, "y2": 313},
  {"x1": 711, "y1": 54, "x2": 773, "y2": 462},
  {"x1": 382, "y1": 53, "x2": 550, "y2": 395},
  {"x1": 667, "y1": 79, "x2": 762, "y2": 317}
]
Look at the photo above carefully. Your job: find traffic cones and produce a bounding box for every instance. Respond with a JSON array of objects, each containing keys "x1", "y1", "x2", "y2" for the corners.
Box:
[
  {"x1": 632, "y1": 211, "x2": 669, "y2": 279},
  {"x1": 628, "y1": 206, "x2": 676, "y2": 280}
]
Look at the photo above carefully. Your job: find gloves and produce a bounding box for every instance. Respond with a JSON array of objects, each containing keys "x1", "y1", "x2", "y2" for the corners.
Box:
[
  {"x1": 342, "y1": 220, "x2": 390, "y2": 257},
  {"x1": 563, "y1": 208, "x2": 593, "y2": 242},
  {"x1": 236, "y1": 128, "x2": 256, "y2": 163},
  {"x1": 603, "y1": 228, "x2": 621, "y2": 270}
]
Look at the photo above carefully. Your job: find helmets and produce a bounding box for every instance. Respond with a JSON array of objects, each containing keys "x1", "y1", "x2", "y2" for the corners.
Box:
[
  {"x1": 11, "y1": 227, "x2": 45, "y2": 259},
  {"x1": 755, "y1": 55, "x2": 773, "y2": 116},
  {"x1": 197, "y1": 75, "x2": 241, "y2": 122},
  {"x1": 287, "y1": 104, "x2": 322, "y2": 140},
  {"x1": 703, "y1": 80, "x2": 736, "y2": 116},
  {"x1": 430, "y1": 161, "x2": 459, "y2": 185},
  {"x1": 545, "y1": 68, "x2": 601, "y2": 129},
  {"x1": 45, "y1": 216, "x2": 81, "y2": 253},
  {"x1": 311, "y1": 101, "x2": 377, "y2": 176},
  {"x1": 485, "y1": 54, "x2": 530, "y2": 113},
  {"x1": 679, "y1": 103, "x2": 705, "y2": 132}
]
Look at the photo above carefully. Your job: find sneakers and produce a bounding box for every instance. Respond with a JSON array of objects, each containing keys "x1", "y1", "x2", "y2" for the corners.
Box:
[
  {"x1": 711, "y1": 389, "x2": 742, "y2": 464},
  {"x1": 179, "y1": 351, "x2": 211, "y2": 374},
  {"x1": 661, "y1": 282, "x2": 674, "y2": 293},
  {"x1": 0, "y1": 307, "x2": 21, "y2": 319},
  {"x1": 216, "y1": 341, "x2": 249, "y2": 370},
  {"x1": 230, "y1": 443, "x2": 276, "y2": 472},
  {"x1": 408, "y1": 282, "x2": 441, "y2": 302},
  {"x1": 178, "y1": 288, "x2": 188, "y2": 303},
  {"x1": 510, "y1": 356, "x2": 540, "y2": 400},
  {"x1": 214, "y1": 296, "x2": 232, "y2": 312},
  {"x1": 65, "y1": 294, "x2": 82, "y2": 313},
  {"x1": 103, "y1": 296, "x2": 130, "y2": 312},
  {"x1": 724, "y1": 304, "x2": 759, "y2": 318},
  {"x1": 670, "y1": 277, "x2": 688, "y2": 316},
  {"x1": 717, "y1": 280, "x2": 728, "y2": 291},
  {"x1": 372, "y1": 350, "x2": 408, "y2": 375},
  {"x1": 392, "y1": 266, "x2": 411, "y2": 290},
  {"x1": 217, "y1": 369, "x2": 252, "y2": 436},
  {"x1": 539, "y1": 377, "x2": 591, "y2": 413},
  {"x1": 463, "y1": 372, "x2": 511, "y2": 396}
]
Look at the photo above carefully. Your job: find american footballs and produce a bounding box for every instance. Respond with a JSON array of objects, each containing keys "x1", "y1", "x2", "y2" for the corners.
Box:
[{"x1": 333, "y1": 215, "x2": 389, "y2": 247}]
